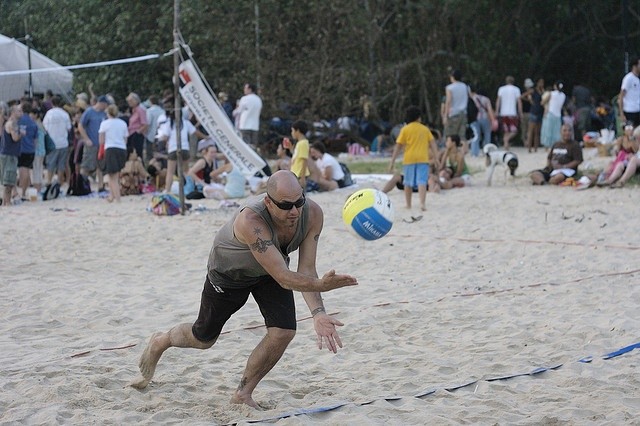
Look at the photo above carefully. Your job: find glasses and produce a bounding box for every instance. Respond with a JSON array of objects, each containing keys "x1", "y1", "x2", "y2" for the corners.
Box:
[{"x1": 267, "y1": 187, "x2": 306, "y2": 210}]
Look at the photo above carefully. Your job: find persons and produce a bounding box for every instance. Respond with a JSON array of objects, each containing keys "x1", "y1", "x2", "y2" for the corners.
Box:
[
  {"x1": 562, "y1": 82, "x2": 615, "y2": 148},
  {"x1": 186, "y1": 139, "x2": 247, "y2": 200},
  {"x1": 443, "y1": 68, "x2": 471, "y2": 155},
  {"x1": 270, "y1": 118, "x2": 345, "y2": 192},
  {"x1": 337, "y1": 89, "x2": 357, "y2": 135},
  {"x1": 494, "y1": 75, "x2": 525, "y2": 150},
  {"x1": 437, "y1": 134, "x2": 472, "y2": 189},
  {"x1": 539, "y1": 80, "x2": 567, "y2": 151},
  {"x1": 378, "y1": 130, "x2": 444, "y2": 194},
  {"x1": 388, "y1": 105, "x2": 440, "y2": 211},
  {"x1": 248, "y1": 144, "x2": 272, "y2": 178},
  {"x1": 345, "y1": 142, "x2": 367, "y2": 156},
  {"x1": 0, "y1": 82, "x2": 211, "y2": 203},
  {"x1": 137, "y1": 169, "x2": 358, "y2": 415},
  {"x1": 217, "y1": 90, "x2": 233, "y2": 121},
  {"x1": 529, "y1": 123, "x2": 583, "y2": 185},
  {"x1": 617, "y1": 57, "x2": 640, "y2": 128},
  {"x1": 596, "y1": 124, "x2": 640, "y2": 188},
  {"x1": 519, "y1": 78, "x2": 535, "y2": 147},
  {"x1": 265, "y1": 107, "x2": 335, "y2": 146},
  {"x1": 359, "y1": 102, "x2": 382, "y2": 147},
  {"x1": 541, "y1": 84, "x2": 552, "y2": 117},
  {"x1": 370, "y1": 128, "x2": 392, "y2": 156},
  {"x1": 518, "y1": 78, "x2": 545, "y2": 153},
  {"x1": 467, "y1": 81, "x2": 495, "y2": 159},
  {"x1": 232, "y1": 81, "x2": 263, "y2": 152},
  {"x1": 441, "y1": 94, "x2": 446, "y2": 146}
]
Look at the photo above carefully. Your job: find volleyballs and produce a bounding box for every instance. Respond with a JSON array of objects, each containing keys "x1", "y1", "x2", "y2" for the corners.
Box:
[{"x1": 341, "y1": 188, "x2": 395, "y2": 241}]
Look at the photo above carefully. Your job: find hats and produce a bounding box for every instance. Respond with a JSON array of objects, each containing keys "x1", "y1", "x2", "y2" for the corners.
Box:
[{"x1": 97, "y1": 95, "x2": 105, "y2": 101}]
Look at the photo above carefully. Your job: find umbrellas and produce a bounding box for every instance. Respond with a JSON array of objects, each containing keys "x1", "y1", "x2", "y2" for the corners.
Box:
[{"x1": 0, "y1": 33, "x2": 75, "y2": 102}]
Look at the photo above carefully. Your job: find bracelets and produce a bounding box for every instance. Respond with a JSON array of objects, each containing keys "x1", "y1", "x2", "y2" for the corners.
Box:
[{"x1": 311, "y1": 307, "x2": 325, "y2": 318}]
